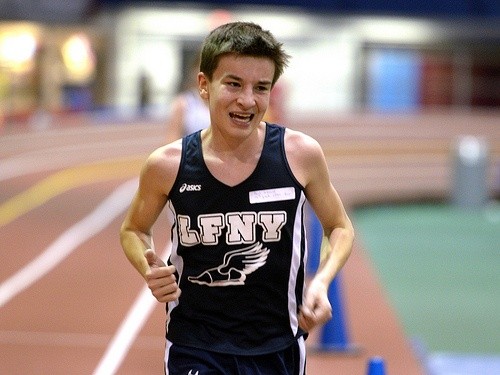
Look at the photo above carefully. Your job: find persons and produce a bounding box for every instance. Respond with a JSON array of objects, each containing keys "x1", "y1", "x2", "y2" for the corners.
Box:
[{"x1": 120, "y1": 22, "x2": 355, "y2": 375}]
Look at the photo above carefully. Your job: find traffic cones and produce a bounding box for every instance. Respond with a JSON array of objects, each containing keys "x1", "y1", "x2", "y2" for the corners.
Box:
[
  {"x1": 366, "y1": 356, "x2": 387, "y2": 375},
  {"x1": 307, "y1": 275, "x2": 363, "y2": 354},
  {"x1": 301, "y1": 201, "x2": 327, "y2": 275}
]
